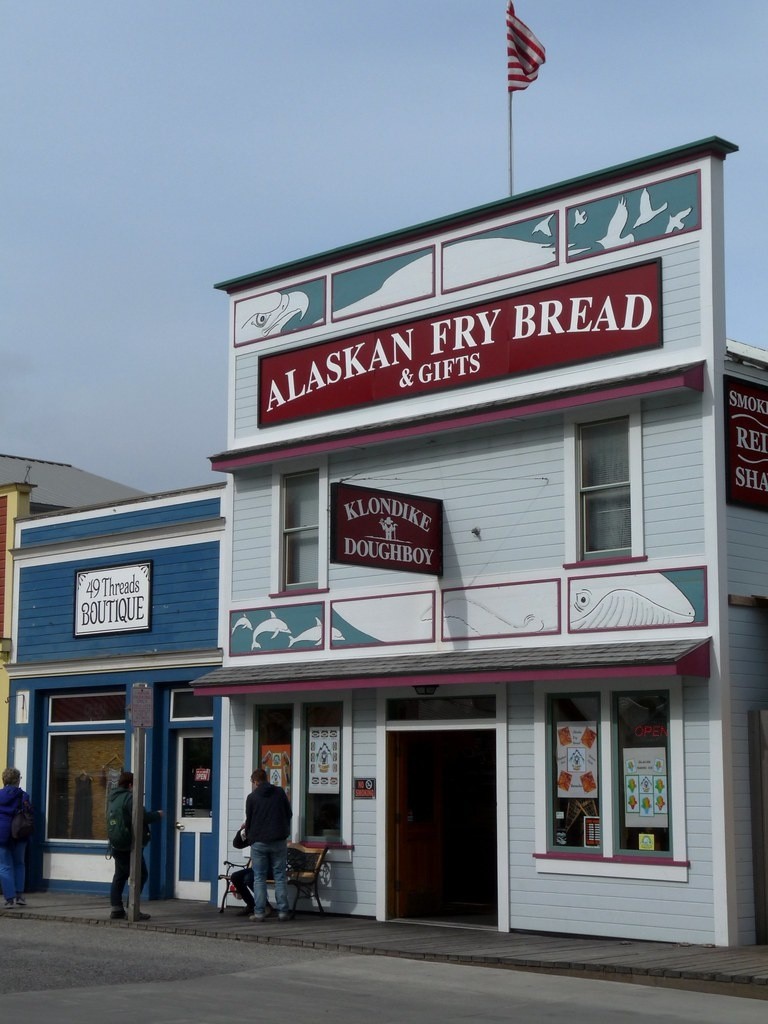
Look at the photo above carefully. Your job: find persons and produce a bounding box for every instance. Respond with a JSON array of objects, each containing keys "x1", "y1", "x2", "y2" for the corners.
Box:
[
  {"x1": 105, "y1": 771, "x2": 165, "y2": 921},
  {"x1": 229, "y1": 820, "x2": 279, "y2": 919},
  {"x1": 239, "y1": 769, "x2": 293, "y2": 921},
  {"x1": 0, "y1": 768, "x2": 34, "y2": 908}
]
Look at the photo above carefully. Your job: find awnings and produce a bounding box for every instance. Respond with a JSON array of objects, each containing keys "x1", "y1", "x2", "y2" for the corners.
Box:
[{"x1": 186, "y1": 636, "x2": 712, "y2": 698}]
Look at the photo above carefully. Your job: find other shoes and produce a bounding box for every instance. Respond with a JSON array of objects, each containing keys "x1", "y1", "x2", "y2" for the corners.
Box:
[
  {"x1": 110, "y1": 909, "x2": 127, "y2": 920},
  {"x1": 1, "y1": 900, "x2": 16, "y2": 908},
  {"x1": 265, "y1": 908, "x2": 277, "y2": 917},
  {"x1": 126, "y1": 911, "x2": 151, "y2": 921},
  {"x1": 237, "y1": 905, "x2": 254, "y2": 916},
  {"x1": 250, "y1": 913, "x2": 266, "y2": 922},
  {"x1": 15, "y1": 896, "x2": 27, "y2": 905},
  {"x1": 279, "y1": 912, "x2": 295, "y2": 921}
]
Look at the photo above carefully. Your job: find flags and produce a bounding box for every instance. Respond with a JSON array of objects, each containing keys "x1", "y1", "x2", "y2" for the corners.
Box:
[{"x1": 505, "y1": 0, "x2": 546, "y2": 93}]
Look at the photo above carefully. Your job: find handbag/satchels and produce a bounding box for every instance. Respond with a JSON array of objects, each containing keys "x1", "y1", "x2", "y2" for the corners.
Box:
[{"x1": 11, "y1": 790, "x2": 35, "y2": 840}]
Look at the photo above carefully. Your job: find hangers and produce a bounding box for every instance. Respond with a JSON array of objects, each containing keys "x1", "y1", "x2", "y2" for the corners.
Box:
[
  {"x1": 74, "y1": 768, "x2": 93, "y2": 782},
  {"x1": 101, "y1": 748, "x2": 126, "y2": 770}
]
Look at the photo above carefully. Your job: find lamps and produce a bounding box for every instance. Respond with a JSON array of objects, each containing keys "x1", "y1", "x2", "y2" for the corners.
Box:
[{"x1": 411, "y1": 685, "x2": 439, "y2": 695}]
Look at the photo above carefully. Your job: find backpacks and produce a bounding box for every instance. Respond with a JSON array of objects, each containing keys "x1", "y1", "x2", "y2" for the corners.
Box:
[{"x1": 107, "y1": 792, "x2": 132, "y2": 848}]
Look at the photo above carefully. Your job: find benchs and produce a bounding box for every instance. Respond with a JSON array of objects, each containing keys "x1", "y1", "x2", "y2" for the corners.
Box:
[{"x1": 217, "y1": 839, "x2": 332, "y2": 920}]
